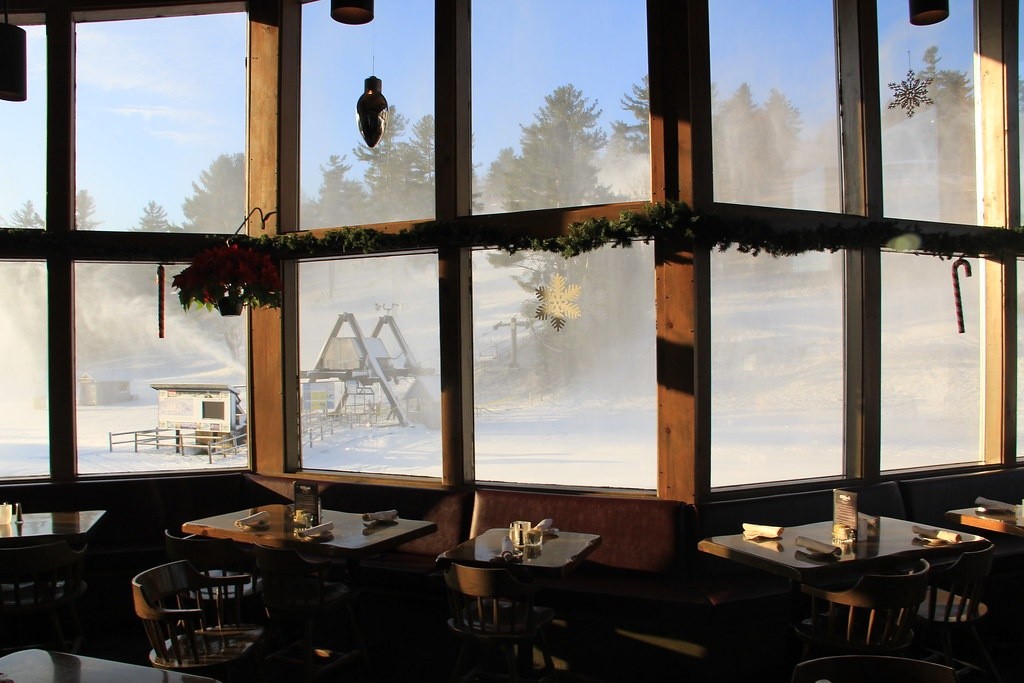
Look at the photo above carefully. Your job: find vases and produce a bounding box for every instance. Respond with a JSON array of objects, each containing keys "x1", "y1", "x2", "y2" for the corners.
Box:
[{"x1": 213, "y1": 286, "x2": 243, "y2": 318}]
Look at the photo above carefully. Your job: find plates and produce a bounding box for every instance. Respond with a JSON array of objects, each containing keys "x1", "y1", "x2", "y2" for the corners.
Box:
[
  {"x1": 293, "y1": 528, "x2": 334, "y2": 540},
  {"x1": 531, "y1": 528, "x2": 559, "y2": 535},
  {"x1": 488, "y1": 549, "x2": 524, "y2": 558},
  {"x1": 799, "y1": 547, "x2": 824, "y2": 556},
  {"x1": 245, "y1": 521, "x2": 271, "y2": 527},
  {"x1": 917, "y1": 535, "x2": 946, "y2": 543},
  {"x1": 374, "y1": 516, "x2": 399, "y2": 522}
]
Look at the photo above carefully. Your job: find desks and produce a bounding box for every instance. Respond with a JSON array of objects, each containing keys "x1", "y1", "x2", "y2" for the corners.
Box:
[
  {"x1": 432, "y1": 527, "x2": 602, "y2": 683},
  {"x1": 697, "y1": 515, "x2": 991, "y2": 683},
  {"x1": 946, "y1": 504, "x2": 1024, "y2": 683},
  {"x1": 181, "y1": 504, "x2": 438, "y2": 683},
  {"x1": 0, "y1": 510, "x2": 108, "y2": 651}
]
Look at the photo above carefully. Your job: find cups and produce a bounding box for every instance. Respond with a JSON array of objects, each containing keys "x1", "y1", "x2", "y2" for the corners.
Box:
[
  {"x1": 0, "y1": 504, "x2": 12, "y2": 524},
  {"x1": 525, "y1": 546, "x2": 542, "y2": 559},
  {"x1": 835, "y1": 528, "x2": 856, "y2": 542},
  {"x1": 526, "y1": 530, "x2": 542, "y2": 546},
  {"x1": 510, "y1": 521, "x2": 531, "y2": 547}
]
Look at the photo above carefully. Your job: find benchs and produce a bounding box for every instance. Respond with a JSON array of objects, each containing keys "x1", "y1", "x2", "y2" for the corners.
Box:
[
  {"x1": 689, "y1": 479, "x2": 956, "y2": 630},
  {"x1": 243, "y1": 474, "x2": 472, "y2": 603},
  {"x1": 465, "y1": 490, "x2": 695, "y2": 626},
  {"x1": 898, "y1": 466, "x2": 1024, "y2": 595},
  {"x1": 0, "y1": 471, "x2": 242, "y2": 580}
]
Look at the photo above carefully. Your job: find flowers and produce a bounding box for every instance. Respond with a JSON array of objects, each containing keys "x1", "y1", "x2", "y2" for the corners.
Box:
[{"x1": 168, "y1": 243, "x2": 286, "y2": 316}]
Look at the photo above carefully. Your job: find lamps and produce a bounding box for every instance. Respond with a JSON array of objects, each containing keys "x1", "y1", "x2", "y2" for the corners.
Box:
[
  {"x1": 909, "y1": 0, "x2": 950, "y2": 26},
  {"x1": 356, "y1": 19, "x2": 388, "y2": 149},
  {"x1": 330, "y1": 0, "x2": 374, "y2": 25}
]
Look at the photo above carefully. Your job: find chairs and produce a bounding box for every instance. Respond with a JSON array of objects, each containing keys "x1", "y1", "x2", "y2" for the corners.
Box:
[{"x1": 0, "y1": 539, "x2": 999, "y2": 683}]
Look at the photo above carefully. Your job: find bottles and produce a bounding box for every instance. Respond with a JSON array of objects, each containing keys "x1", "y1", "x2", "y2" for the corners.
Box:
[{"x1": 15, "y1": 503, "x2": 22, "y2": 523}]
